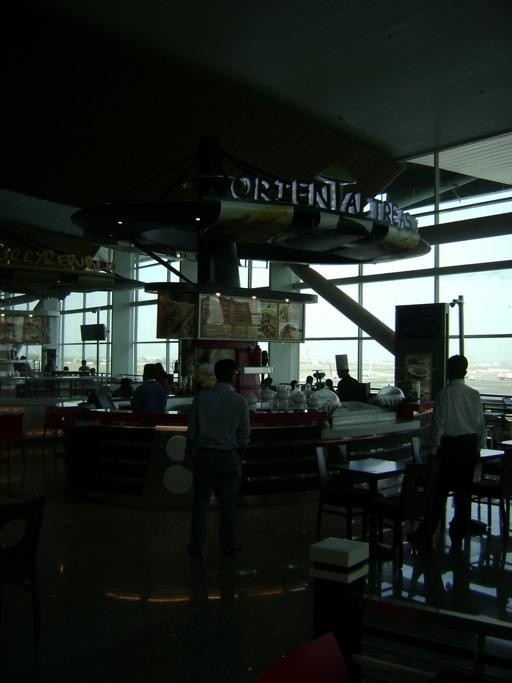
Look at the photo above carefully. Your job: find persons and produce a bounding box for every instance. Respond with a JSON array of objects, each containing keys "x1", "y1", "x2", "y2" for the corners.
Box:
[
  {"x1": 156, "y1": 362, "x2": 171, "y2": 395},
  {"x1": 130, "y1": 364, "x2": 167, "y2": 426},
  {"x1": 17, "y1": 356, "x2": 32, "y2": 372},
  {"x1": 260, "y1": 368, "x2": 366, "y2": 402},
  {"x1": 45, "y1": 357, "x2": 57, "y2": 376},
  {"x1": 79, "y1": 360, "x2": 90, "y2": 397},
  {"x1": 406, "y1": 355, "x2": 485, "y2": 560},
  {"x1": 90, "y1": 368, "x2": 96, "y2": 376},
  {"x1": 183, "y1": 358, "x2": 251, "y2": 556}
]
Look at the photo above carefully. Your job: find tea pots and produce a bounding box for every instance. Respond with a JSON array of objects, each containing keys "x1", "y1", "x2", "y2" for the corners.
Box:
[{"x1": 240, "y1": 385, "x2": 323, "y2": 409}]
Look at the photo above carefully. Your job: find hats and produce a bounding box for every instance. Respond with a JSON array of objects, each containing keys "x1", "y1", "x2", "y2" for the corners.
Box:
[{"x1": 336, "y1": 354, "x2": 348, "y2": 371}]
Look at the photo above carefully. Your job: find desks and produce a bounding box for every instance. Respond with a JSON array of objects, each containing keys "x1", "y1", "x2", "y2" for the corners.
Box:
[{"x1": 33, "y1": 371, "x2": 111, "y2": 399}]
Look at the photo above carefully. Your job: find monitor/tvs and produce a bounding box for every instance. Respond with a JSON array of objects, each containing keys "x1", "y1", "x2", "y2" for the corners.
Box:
[
  {"x1": 81, "y1": 324, "x2": 105, "y2": 340},
  {"x1": 92, "y1": 392, "x2": 116, "y2": 409}
]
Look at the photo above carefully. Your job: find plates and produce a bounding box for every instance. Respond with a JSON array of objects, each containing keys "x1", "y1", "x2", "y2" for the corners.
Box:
[{"x1": 407, "y1": 365, "x2": 430, "y2": 377}]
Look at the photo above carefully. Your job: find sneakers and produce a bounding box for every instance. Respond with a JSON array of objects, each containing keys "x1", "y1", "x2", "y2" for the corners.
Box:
[{"x1": 187, "y1": 539, "x2": 244, "y2": 555}]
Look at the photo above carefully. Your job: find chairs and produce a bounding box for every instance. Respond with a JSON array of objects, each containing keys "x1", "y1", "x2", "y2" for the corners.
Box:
[
  {"x1": 42, "y1": 407, "x2": 76, "y2": 469},
  {"x1": 1, "y1": 495, "x2": 47, "y2": 666},
  {"x1": 0, "y1": 409, "x2": 26, "y2": 489},
  {"x1": 313, "y1": 436, "x2": 511, "y2": 568}
]
